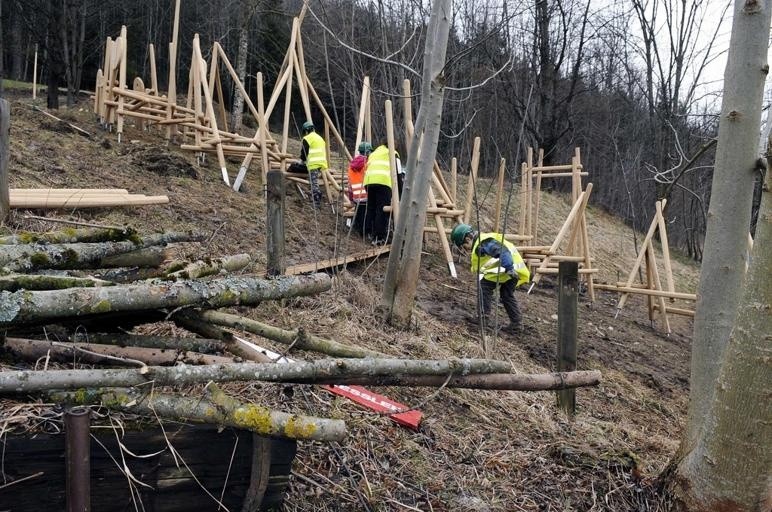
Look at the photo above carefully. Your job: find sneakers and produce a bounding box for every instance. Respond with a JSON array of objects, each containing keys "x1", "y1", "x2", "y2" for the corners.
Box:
[
  {"x1": 467, "y1": 316, "x2": 489, "y2": 327},
  {"x1": 502, "y1": 321, "x2": 525, "y2": 333}
]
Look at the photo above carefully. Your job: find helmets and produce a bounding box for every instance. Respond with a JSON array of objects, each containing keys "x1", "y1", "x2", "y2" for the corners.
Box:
[
  {"x1": 450, "y1": 223, "x2": 472, "y2": 247},
  {"x1": 302, "y1": 121, "x2": 314, "y2": 130},
  {"x1": 358, "y1": 142, "x2": 372, "y2": 151}
]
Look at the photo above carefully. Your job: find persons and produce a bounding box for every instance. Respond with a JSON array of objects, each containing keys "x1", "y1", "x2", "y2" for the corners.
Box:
[
  {"x1": 363, "y1": 135, "x2": 401, "y2": 246},
  {"x1": 295, "y1": 121, "x2": 329, "y2": 210},
  {"x1": 450, "y1": 223, "x2": 531, "y2": 334},
  {"x1": 348, "y1": 141, "x2": 375, "y2": 240}
]
